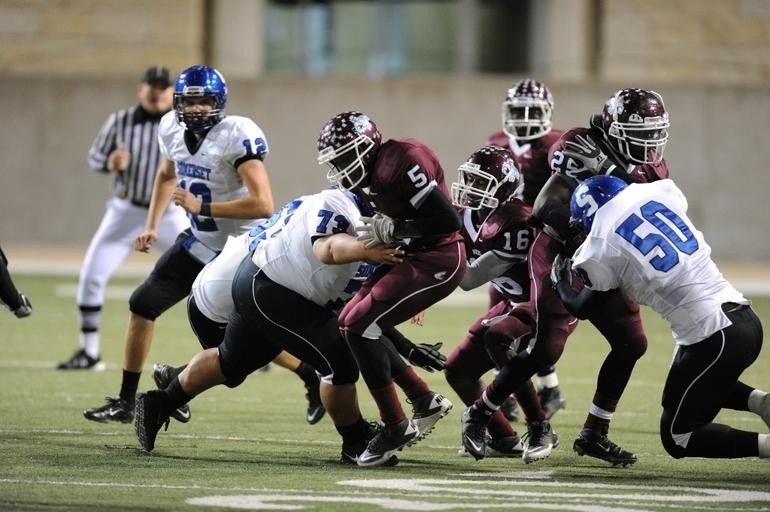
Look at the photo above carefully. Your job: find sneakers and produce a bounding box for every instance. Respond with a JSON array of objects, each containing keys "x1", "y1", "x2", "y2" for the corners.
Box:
[
  {"x1": 573, "y1": 427, "x2": 637, "y2": 468},
  {"x1": 151, "y1": 362, "x2": 191, "y2": 422},
  {"x1": 459, "y1": 398, "x2": 560, "y2": 464},
  {"x1": 82, "y1": 396, "x2": 134, "y2": 424},
  {"x1": 539, "y1": 388, "x2": 567, "y2": 421},
  {"x1": 134, "y1": 389, "x2": 170, "y2": 453},
  {"x1": 56, "y1": 348, "x2": 106, "y2": 371},
  {"x1": 341, "y1": 392, "x2": 453, "y2": 468},
  {"x1": 305, "y1": 366, "x2": 327, "y2": 424}
]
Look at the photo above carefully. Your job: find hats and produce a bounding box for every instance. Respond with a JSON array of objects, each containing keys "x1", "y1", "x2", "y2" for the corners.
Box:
[{"x1": 142, "y1": 65, "x2": 173, "y2": 83}]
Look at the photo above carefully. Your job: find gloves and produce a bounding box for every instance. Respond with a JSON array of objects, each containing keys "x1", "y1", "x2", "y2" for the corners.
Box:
[
  {"x1": 561, "y1": 134, "x2": 618, "y2": 179},
  {"x1": 354, "y1": 211, "x2": 394, "y2": 248},
  {"x1": 405, "y1": 342, "x2": 448, "y2": 374}
]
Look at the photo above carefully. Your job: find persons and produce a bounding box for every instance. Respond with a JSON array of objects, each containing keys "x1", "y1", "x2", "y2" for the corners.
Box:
[
  {"x1": 485, "y1": 81, "x2": 570, "y2": 423},
  {"x1": 153, "y1": 192, "x2": 447, "y2": 423},
  {"x1": 450, "y1": 145, "x2": 559, "y2": 464},
  {"x1": 550, "y1": 174, "x2": 770, "y2": 459},
  {"x1": 56, "y1": 67, "x2": 272, "y2": 371},
  {"x1": 84, "y1": 66, "x2": 326, "y2": 424},
  {"x1": 135, "y1": 188, "x2": 406, "y2": 468},
  {"x1": 460, "y1": 88, "x2": 668, "y2": 468},
  {"x1": 0, "y1": 249, "x2": 32, "y2": 318},
  {"x1": 317, "y1": 110, "x2": 467, "y2": 466}
]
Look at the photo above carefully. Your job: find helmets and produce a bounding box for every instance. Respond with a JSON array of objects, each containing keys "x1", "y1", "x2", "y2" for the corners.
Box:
[
  {"x1": 570, "y1": 174, "x2": 629, "y2": 231},
  {"x1": 501, "y1": 77, "x2": 554, "y2": 138},
  {"x1": 173, "y1": 64, "x2": 228, "y2": 131},
  {"x1": 449, "y1": 144, "x2": 521, "y2": 208},
  {"x1": 600, "y1": 87, "x2": 671, "y2": 165},
  {"x1": 314, "y1": 110, "x2": 385, "y2": 194}
]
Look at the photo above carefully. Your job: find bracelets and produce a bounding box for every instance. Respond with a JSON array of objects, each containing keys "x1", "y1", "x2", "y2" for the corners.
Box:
[{"x1": 200, "y1": 203, "x2": 210, "y2": 217}]
